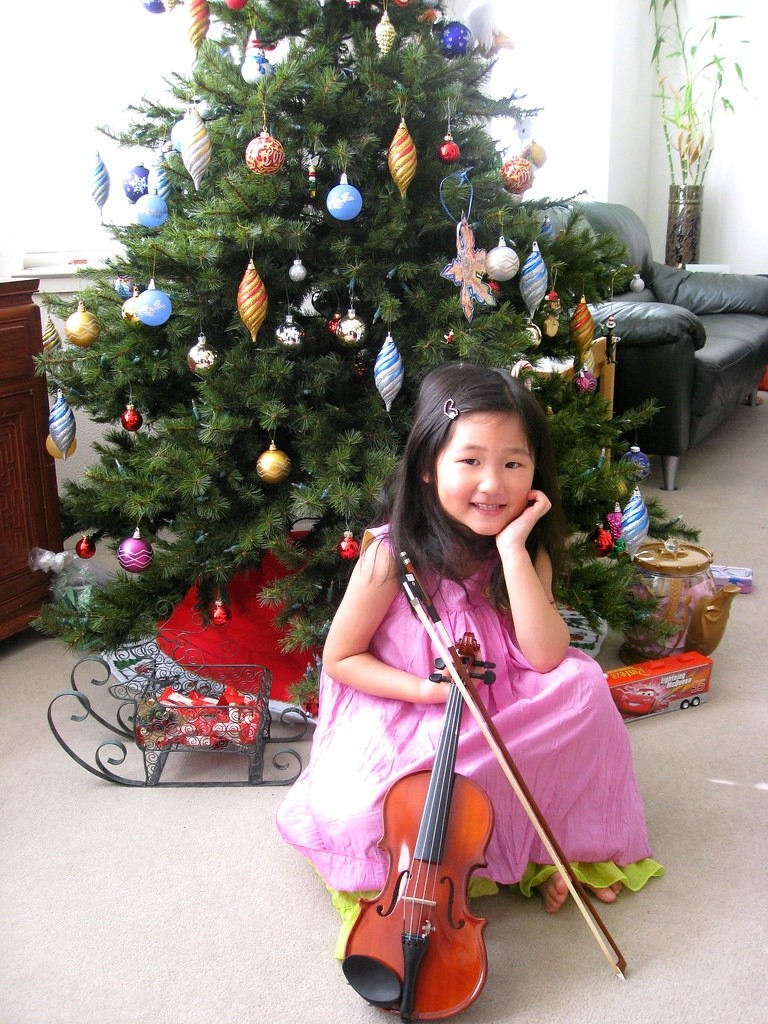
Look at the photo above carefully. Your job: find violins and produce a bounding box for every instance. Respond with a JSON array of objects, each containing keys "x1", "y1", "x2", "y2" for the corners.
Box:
[{"x1": 338, "y1": 630, "x2": 497, "y2": 1024}]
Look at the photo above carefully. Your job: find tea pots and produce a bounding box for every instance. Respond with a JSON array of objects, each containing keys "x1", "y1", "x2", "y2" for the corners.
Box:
[{"x1": 619, "y1": 538, "x2": 740, "y2": 664}]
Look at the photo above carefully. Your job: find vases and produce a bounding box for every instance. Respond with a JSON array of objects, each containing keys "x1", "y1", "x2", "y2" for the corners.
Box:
[{"x1": 664, "y1": 186, "x2": 705, "y2": 271}]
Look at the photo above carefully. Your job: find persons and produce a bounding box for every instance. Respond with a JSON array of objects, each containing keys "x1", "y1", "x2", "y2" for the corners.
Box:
[{"x1": 274, "y1": 364, "x2": 665, "y2": 913}]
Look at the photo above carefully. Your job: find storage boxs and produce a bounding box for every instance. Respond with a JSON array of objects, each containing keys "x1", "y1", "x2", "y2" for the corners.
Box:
[{"x1": 710, "y1": 564, "x2": 754, "y2": 594}]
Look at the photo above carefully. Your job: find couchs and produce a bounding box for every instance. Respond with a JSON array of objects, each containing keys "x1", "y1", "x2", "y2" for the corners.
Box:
[{"x1": 536, "y1": 201, "x2": 768, "y2": 490}]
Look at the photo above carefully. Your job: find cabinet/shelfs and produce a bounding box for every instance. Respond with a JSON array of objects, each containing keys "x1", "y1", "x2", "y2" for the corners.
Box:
[{"x1": 0, "y1": 276, "x2": 66, "y2": 640}]
